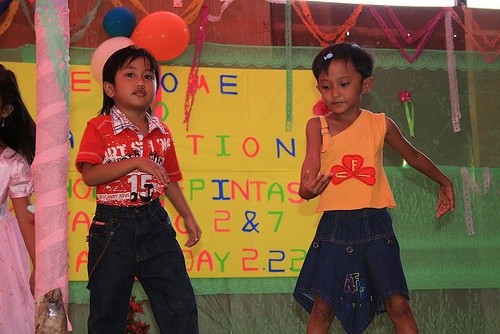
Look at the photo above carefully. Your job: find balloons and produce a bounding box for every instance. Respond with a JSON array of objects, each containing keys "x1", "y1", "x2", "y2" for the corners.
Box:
[{"x1": 90, "y1": 8, "x2": 189, "y2": 85}]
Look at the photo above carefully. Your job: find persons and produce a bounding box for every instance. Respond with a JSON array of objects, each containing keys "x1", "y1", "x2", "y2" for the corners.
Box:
[
  {"x1": 292, "y1": 43, "x2": 454, "y2": 334},
  {"x1": 0, "y1": 63, "x2": 36, "y2": 334},
  {"x1": 75, "y1": 45, "x2": 202, "y2": 334}
]
